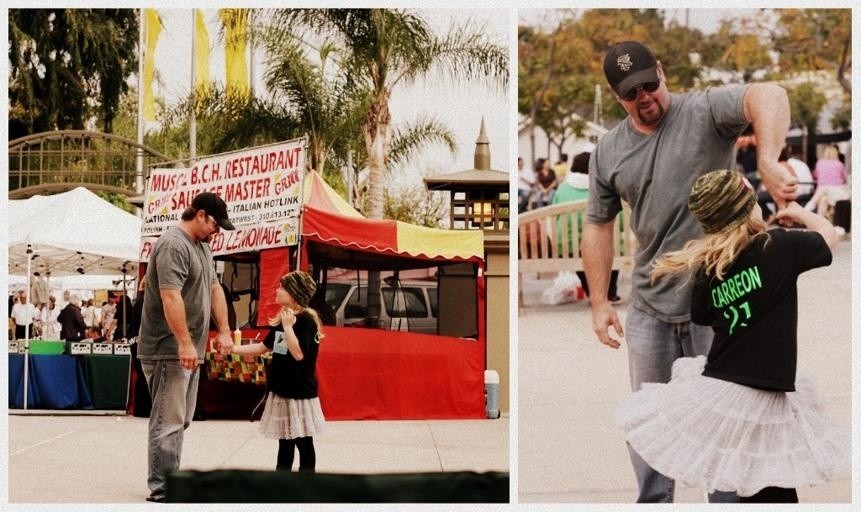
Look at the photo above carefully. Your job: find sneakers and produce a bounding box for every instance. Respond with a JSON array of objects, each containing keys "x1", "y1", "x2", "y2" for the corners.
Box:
[{"x1": 608, "y1": 294, "x2": 620, "y2": 301}]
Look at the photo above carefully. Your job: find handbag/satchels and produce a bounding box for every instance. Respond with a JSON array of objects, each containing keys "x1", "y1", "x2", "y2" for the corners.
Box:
[{"x1": 85, "y1": 327, "x2": 101, "y2": 341}]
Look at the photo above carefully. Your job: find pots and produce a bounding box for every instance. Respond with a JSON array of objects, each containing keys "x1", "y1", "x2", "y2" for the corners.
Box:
[{"x1": 241, "y1": 339, "x2": 261, "y2": 345}]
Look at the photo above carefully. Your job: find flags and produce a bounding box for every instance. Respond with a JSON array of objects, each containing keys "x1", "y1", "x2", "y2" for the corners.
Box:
[
  {"x1": 141, "y1": 9, "x2": 163, "y2": 123},
  {"x1": 221, "y1": 9, "x2": 251, "y2": 104},
  {"x1": 194, "y1": 9, "x2": 212, "y2": 102}
]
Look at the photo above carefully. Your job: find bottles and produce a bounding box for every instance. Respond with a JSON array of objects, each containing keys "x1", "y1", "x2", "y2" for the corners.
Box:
[{"x1": 233, "y1": 327, "x2": 242, "y2": 346}]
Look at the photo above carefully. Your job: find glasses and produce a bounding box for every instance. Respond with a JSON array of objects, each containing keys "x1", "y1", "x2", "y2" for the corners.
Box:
[
  {"x1": 621, "y1": 83, "x2": 657, "y2": 100},
  {"x1": 208, "y1": 216, "x2": 221, "y2": 227}
]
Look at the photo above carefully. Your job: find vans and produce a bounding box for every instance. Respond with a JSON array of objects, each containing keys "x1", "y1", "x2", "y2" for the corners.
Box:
[{"x1": 315, "y1": 278, "x2": 439, "y2": 336}]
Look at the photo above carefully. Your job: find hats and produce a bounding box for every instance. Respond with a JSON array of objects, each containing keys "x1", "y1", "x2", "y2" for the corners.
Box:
[
  {"x1": 107, "y1": 294, "x2": 116, "y2": 297},
  {"x1": 279, "y1": 270, "x2": 316, "y2": 308},
  {"x1": 688, "y1": 171, "x2": 759, "y2": 234},
  {"x1": 190, "y1": 193, "x2": 235, "y2": 230},
  {"x1": 605, "y1": 40, "x2": 659, "y2": 97}
]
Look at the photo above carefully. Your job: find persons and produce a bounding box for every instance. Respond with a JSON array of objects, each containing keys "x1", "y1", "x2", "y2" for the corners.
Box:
[
  {"x1": 127, "y1": 274, "x2": 150, "y2": 418},
  {"x1": 211, "y1": 271, "x2": 327, "y2": 474},
  {"x1": 732, "y1": 120, "x2": 852, "y2": 240},
  {"x1": 136, "y1": 191, "x2": 235, "y2": 501},
  {"x1": 518, "y1": 134, "x2": 625, "y2": 306},
  {"x1": 579, "y1": 40, "x2": 799, "y2": 503},
  {"x1": 8, "y1": 289, "x2": 153, "y2": 342},
  {"x1": 614, "y1": 168, "x2": 843, "y2": 503}
]
